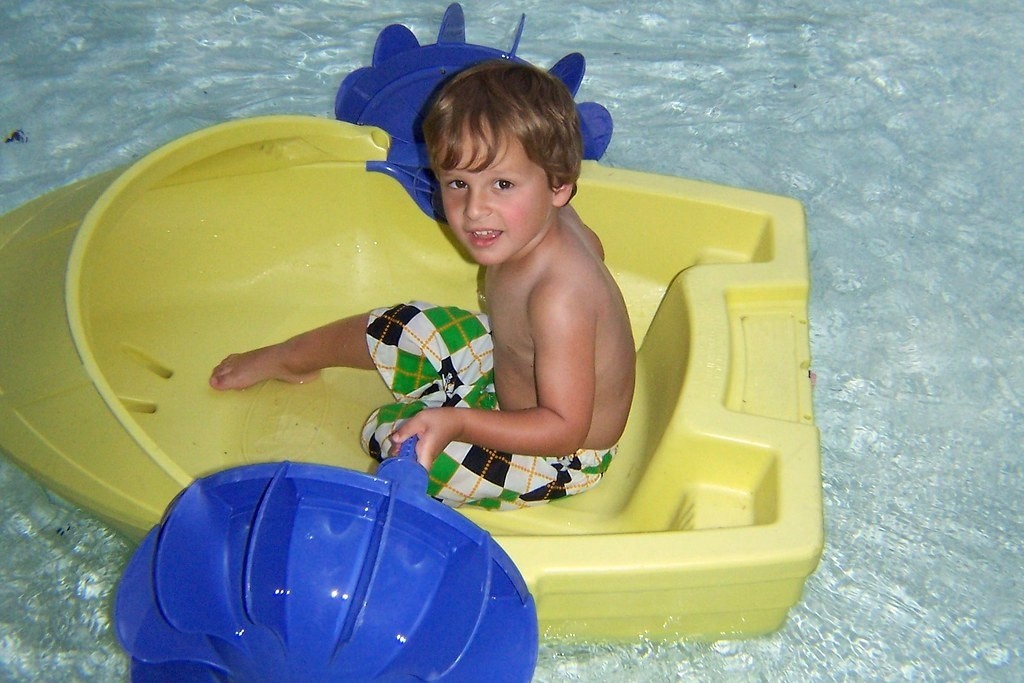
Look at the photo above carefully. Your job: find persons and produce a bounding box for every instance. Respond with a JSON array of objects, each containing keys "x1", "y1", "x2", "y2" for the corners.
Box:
[{"x1": 208, "y1": 60, "x2": 637, "y2": 511}]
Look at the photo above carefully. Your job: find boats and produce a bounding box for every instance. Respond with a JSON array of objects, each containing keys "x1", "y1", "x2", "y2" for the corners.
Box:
[{"x1": 1, "y1": 2, "x2": 824, "y2": 682}]
